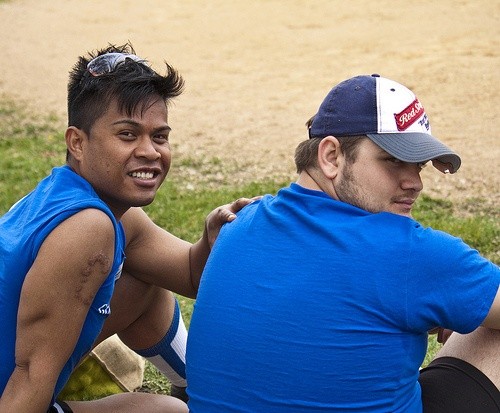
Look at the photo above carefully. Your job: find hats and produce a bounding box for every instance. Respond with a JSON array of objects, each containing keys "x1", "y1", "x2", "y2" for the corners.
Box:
[{"x1": 309, "y1": 74, "x2": 462, "y2": 175}]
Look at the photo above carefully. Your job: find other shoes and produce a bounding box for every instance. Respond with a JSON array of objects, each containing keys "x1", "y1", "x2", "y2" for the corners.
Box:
[{"x1": 170, "y1": 384, "x2": 188, "y2": 402}]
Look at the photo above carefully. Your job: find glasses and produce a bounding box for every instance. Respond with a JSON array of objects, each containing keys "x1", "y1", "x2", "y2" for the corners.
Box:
[{"x1": 66, "y1": 52, "x2": 158, "y2": 114}]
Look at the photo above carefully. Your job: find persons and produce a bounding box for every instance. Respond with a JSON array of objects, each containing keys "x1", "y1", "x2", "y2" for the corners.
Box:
[
  {"x1": 0, "y1": 38, "x2": 263, "y2": 412},
  {"x1": 185, "y1": 72, "x2": 500, "y2": 412}
]
